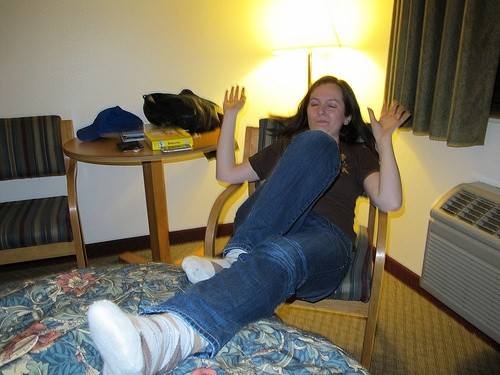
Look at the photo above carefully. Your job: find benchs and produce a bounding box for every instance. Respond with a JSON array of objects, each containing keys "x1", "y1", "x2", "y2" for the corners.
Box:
[{"x1": 0, "y1": 115, "x2": 88, "y2": 270}]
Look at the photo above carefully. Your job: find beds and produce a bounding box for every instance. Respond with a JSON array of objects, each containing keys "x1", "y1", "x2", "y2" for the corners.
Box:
[{"x1": 0, "y1": 262, "x2": 371, "y2": 375}]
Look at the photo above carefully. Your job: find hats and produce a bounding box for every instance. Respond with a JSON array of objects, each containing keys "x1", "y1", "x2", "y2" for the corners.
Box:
[{"x1": 76, "y1": 106, "x2": 144, "y2": 141}]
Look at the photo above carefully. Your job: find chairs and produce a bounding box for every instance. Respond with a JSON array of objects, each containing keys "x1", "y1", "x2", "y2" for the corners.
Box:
[{"x1": 204, "y1": 126, "x2": 388, "y2": 372}]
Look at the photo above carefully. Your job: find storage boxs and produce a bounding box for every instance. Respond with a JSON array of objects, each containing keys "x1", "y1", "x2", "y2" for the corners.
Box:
[{"x1": 143, "y1": 127, "x2": 193, "y2": 151}]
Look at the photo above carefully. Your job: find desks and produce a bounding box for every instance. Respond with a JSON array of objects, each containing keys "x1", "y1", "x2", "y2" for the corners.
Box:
[{"x1": 63, "y1": 122, "x2": 238, "y2": 269}]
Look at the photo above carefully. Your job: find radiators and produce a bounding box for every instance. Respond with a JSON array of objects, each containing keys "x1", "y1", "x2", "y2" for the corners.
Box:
[{"x1": 419, "y1": 183, "x2": 500, "y2": 342}]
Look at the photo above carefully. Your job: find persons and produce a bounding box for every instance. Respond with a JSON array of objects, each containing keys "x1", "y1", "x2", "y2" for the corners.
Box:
[{"x1": 88, "y1": 75, "x2": 411, "y2": 375}]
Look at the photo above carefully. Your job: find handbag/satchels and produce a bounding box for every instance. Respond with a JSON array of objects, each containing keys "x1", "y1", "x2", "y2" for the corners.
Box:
[{"x1": 143, "y1": 90, "x2": 223, "y2": 132}]
